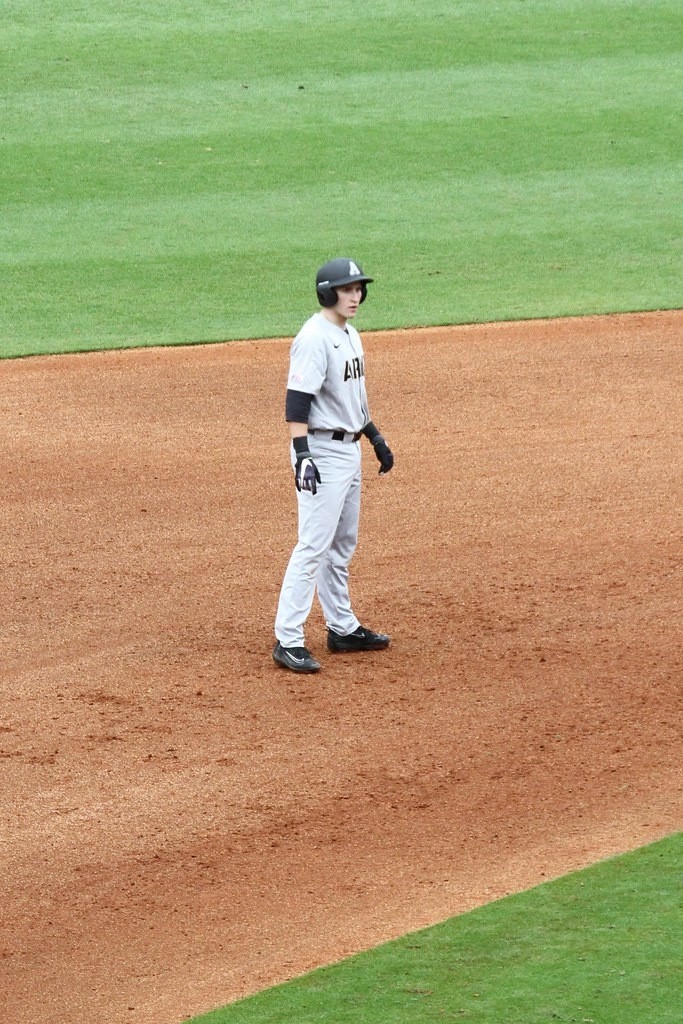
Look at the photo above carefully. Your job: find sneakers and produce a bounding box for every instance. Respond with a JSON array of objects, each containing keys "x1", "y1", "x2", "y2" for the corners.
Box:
[
  {"x1": 327, "y1": 624, "x2": 390, "y2": 653},
  {"x1": 273, "y1": 639, "x2": 321, "y2": 674}
]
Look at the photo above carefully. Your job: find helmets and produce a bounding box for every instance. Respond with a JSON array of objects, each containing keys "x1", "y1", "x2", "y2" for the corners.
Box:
[{"x1": 316, "y1": 258, "x2": 374, "y2": 307}]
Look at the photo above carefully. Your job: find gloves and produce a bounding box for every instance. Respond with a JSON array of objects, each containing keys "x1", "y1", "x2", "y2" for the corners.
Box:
[
  {"x1": 294, "y1": 453, "x2": 321, "y2": 496},
  {"x1": 371, "y1": 435, "x2": 394, "y2": 476}
]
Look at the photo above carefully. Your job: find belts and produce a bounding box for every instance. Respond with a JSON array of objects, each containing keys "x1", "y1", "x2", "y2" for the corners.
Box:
[{"x1": 308, "y1": 428, "x2": 362, "y2": 442}]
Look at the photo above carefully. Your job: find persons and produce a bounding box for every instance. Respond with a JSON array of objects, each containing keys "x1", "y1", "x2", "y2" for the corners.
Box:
[{"x1": 273, "y1": 258, "x2": 395, "y2": 675}]
